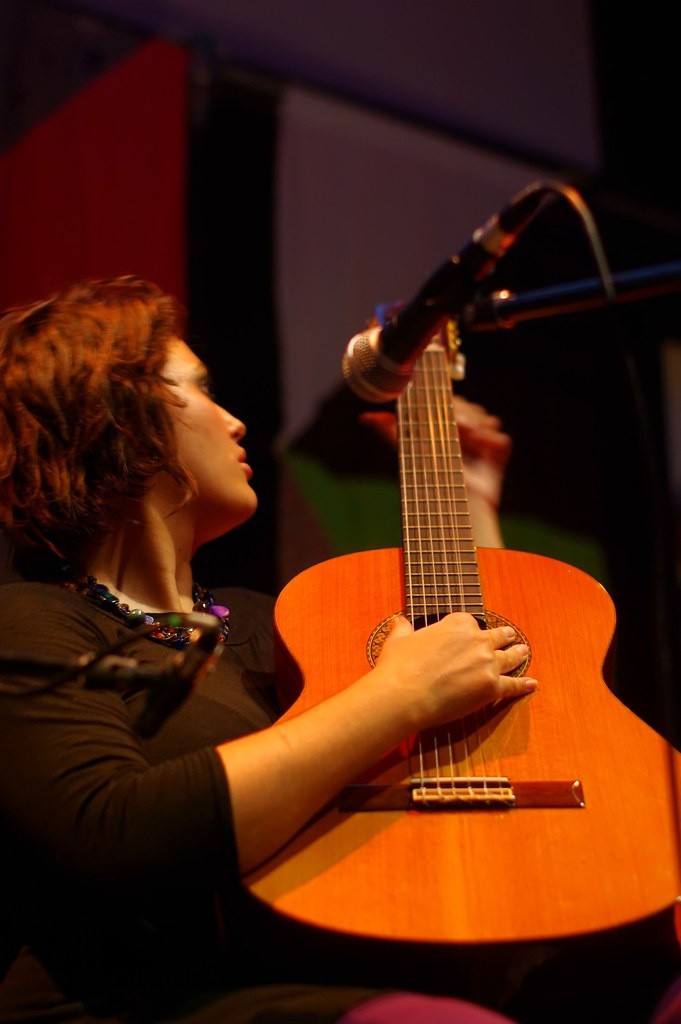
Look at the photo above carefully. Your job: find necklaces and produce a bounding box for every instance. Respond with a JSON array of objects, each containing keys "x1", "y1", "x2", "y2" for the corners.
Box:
[{"x1": 79, "y1": 573, "x2": 232, "y2": 648}]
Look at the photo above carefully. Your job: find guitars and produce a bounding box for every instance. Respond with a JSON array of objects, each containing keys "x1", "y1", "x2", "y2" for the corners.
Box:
[{"x1": 242, "y1": 317, "x2": 679, "y2": 950}]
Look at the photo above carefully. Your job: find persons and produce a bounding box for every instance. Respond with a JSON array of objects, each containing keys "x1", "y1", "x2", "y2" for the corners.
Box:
[{"x1": 0, "y1": 275, "x2": 541, "y2": 1024}]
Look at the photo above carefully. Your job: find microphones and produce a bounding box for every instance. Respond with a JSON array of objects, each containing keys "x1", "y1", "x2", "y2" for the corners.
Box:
[{"x1": 343, "y1": 183, "x2": 548, "y2": 402}]
